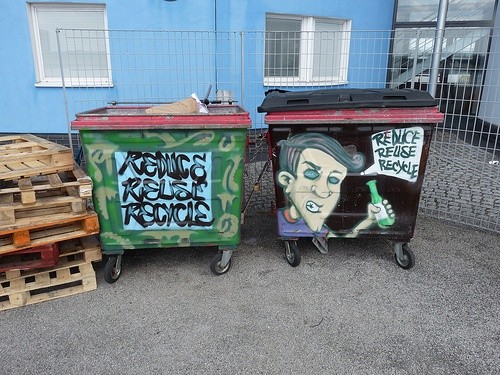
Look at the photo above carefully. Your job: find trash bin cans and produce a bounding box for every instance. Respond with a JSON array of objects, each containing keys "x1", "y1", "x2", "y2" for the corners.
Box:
[
  {"x1": 68, "y1": 98, "x2": 253, "y2": 284},
  {"x1": 256, "y1": 87, "x2": 446, "y2": 271}
]
